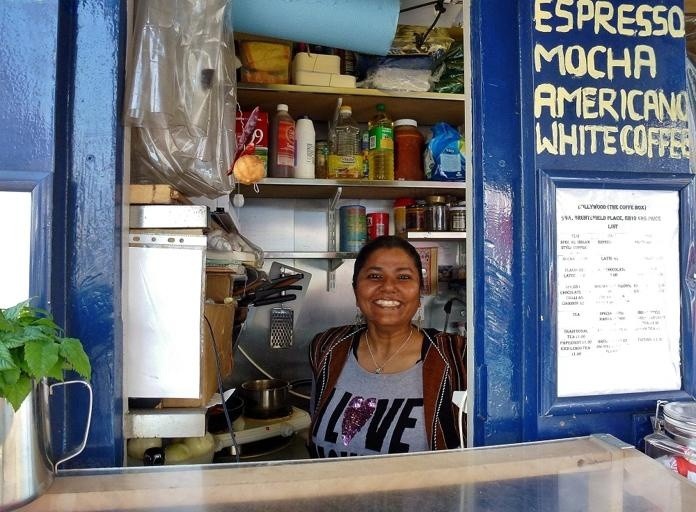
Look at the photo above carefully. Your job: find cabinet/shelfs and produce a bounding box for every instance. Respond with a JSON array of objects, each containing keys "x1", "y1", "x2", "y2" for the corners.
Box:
[{"x1": 206, "y1": 1, "x2": 471, "y2": 296}]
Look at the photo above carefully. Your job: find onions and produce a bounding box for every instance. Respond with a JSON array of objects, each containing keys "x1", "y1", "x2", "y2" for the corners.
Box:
[{"x1": 127, "y1": 432, "x2": 214, "y2": 462}]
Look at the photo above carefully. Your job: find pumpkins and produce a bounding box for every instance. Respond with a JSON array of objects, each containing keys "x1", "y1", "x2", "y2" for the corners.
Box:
[{"x1": 233, "y1": 155, "x2": 264, "y2": 184}]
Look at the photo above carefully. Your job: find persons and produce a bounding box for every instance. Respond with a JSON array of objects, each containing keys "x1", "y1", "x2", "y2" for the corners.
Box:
[{"x1": 305, "y1": 233, "x2": 465, "y2": 458}]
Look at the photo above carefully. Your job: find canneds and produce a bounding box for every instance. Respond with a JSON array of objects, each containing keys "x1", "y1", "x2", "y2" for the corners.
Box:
[
  {"x1": 406, "y1": 207, "x2": 426, "y2": 232},
  {"x1": 448, "y1": 207, "x2": 466, "y2": 232}
]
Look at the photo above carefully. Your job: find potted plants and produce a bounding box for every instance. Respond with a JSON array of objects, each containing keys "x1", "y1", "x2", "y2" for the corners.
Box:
[{"x1": 1, "y1": 301, "x2": 93, "y2": 512}]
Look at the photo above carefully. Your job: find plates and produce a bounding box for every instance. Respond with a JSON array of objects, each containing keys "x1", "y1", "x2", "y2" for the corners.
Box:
[{"x1": 649, "y1": 399, "x2": 694, "y2": 452}]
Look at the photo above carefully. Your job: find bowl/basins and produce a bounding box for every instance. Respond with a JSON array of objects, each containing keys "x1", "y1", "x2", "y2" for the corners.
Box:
[{"x1": 242, "y1": 379, "x2": 294, "y2": 420}]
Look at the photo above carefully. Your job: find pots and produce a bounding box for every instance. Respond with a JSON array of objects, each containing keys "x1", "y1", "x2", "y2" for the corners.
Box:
[{"x1": 235, "y1": 266, "x2": 304, "y2": 324}]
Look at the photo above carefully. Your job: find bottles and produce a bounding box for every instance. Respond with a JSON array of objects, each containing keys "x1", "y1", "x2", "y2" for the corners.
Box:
[{"x1": 271, "y1": 100, "x2": 423, "y2": 181}]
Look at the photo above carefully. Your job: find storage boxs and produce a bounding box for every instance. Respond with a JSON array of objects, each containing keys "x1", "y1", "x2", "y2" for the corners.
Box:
[{"x1": 156, "y1": 274, "x2": 235, "y2": 408}]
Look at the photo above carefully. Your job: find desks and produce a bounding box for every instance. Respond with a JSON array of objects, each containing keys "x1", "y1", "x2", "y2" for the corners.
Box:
[{"x1": 1, "y1": 432, "x2": 695, "y2": 512}]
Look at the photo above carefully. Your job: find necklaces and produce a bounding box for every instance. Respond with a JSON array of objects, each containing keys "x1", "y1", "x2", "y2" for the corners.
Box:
[{"x1": 364, "y1": 330, "x2": 416, "y2": 374}]
